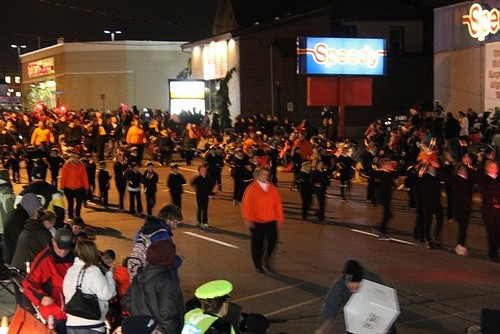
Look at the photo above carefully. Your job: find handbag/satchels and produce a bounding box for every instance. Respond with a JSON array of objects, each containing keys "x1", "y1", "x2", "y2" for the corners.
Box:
[{"x1": 63, "y1": 263, "x2": 101, "y2": 320}]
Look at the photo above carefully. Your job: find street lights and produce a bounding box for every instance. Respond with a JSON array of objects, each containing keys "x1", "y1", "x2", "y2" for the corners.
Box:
[
  {"x1": 7, "y1": 87, "x2": 14, "y2": 107},
  {"x1": 11, "y1": 45, "x2": 26, "y2": 77},
  {"x1": 104, "y1": 30, "x2": 122, "y2": 40}
]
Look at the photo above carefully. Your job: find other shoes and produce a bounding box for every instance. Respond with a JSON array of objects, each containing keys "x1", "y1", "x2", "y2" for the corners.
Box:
[
  {"x1": 425, "y1": 238, "x2": 444, "y2": 250},
  {"x1": 455, "y1": 244, "x2": 470, "y2": 256},
  {"x1": 369, "y1": 225, "x2": 391, "y2": 241}
]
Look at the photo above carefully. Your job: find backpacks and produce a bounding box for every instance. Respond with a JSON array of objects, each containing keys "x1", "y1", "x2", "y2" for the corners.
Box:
[{"x1": 126, "y1": 228, "x2": 168, "y2": 284}]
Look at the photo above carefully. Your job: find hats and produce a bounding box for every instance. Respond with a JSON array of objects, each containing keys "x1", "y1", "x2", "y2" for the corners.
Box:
[
  {"x1": 341, "y1": 259, "x2": 364, "y2": 282},
  {"x1": 121, "y1": 314, "x2": 158, "y2": 334},
  {"x1": 31, "y1": 166, "x2": 45, "y2": 178},
  {"x1": 194, "y1": 279, "x2": 233, "y2": 305},
  {"x1": 55, "y1": 228, "x2": 73, "y2": 250},
  {"x1": 145, "y1": 239, "x2": 176, "y2": 267}
]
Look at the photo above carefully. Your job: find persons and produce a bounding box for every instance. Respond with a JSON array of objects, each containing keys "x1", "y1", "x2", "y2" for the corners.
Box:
[
  {"x1": 0, "y1": 103, "x2": 500, "y2": 334},
  {"x1": 242, "y1": 165, "x2": 284, "y2": 273}
]
